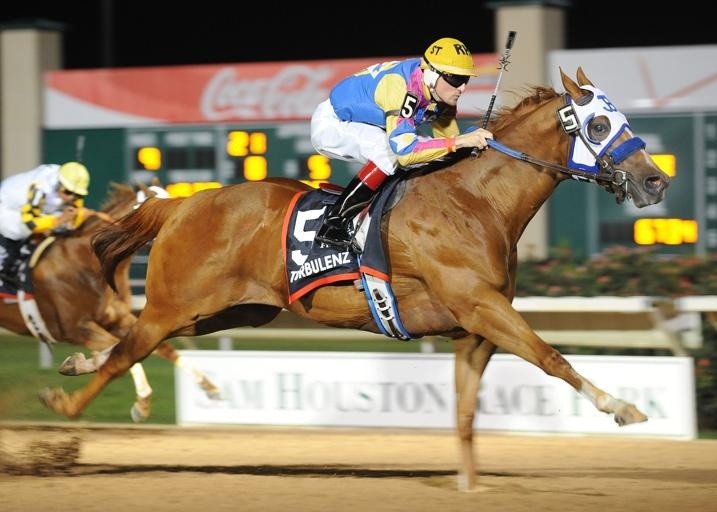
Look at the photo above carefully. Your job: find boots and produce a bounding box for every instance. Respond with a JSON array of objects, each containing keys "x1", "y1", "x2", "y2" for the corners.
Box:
[{"x1": 314, "y1": 158, "x2": 387, "y2": 255}]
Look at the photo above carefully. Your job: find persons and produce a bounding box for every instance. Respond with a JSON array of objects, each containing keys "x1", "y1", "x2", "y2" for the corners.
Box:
[
  {"x1": 1, "y1": 160, "x2": 93, "y2": 298},
  {"x1": 309, "y1": 36, "x2": 495, "y2": 253}
]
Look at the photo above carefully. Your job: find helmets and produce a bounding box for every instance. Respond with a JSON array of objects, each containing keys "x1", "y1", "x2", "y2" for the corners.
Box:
[
  {"x1": 419, "y1": 37, "x2": 479, "y2": 77},
  {"x1": 58, "y1": 161, "x2": 91, "y2": 196}
]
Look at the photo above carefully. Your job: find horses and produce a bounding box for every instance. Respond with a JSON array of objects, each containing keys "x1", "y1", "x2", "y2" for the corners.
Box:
[
  {"x1": 33, "y1": 66, "x2": 673, "y2": 493},
  {"x1": 0, "y1": 175, "x2": 227, "y2": 425}
]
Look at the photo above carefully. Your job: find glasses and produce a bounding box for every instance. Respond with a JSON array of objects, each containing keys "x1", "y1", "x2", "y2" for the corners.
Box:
[
  {"x1": 63, "y1": 189, "x2": 83, "y2": 198},
  {"x1": 440, "y1": 74, "x2": 470, "y2": 88}
]
